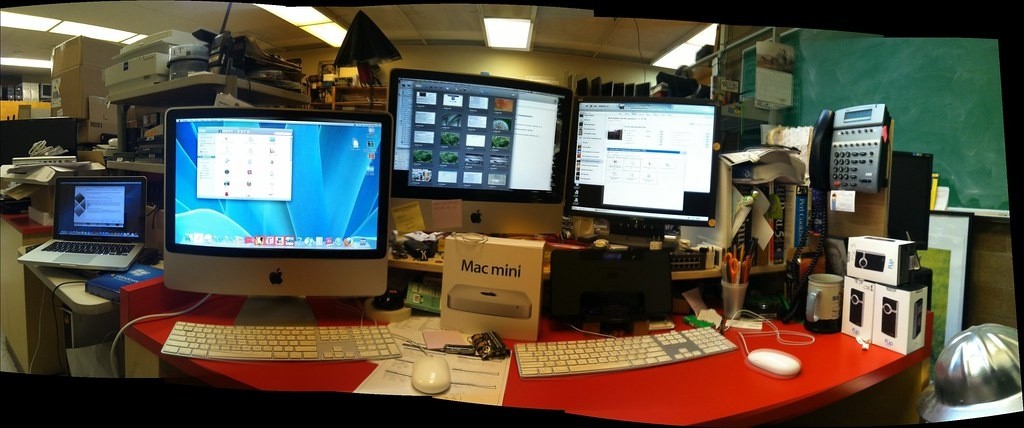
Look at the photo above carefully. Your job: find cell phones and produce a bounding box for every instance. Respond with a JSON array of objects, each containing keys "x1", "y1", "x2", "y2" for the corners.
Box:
[
  {"x1": 849, "y1": 289, "x2": 865, "y2": 327},
  {"x1": 855, "y1": 250, "x2": 886, "y2": 272},
  {"x1": 882, "y1": 297, "x2": 899, "y2": 338},
  {"x1": 913, "y1": 298, "x2": 924, "y2": 340}
]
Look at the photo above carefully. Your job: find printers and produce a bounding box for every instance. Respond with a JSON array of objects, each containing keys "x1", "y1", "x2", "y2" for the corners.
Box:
[{"x1": 104, "y1": 29, "x2": 209, "y2": 92}]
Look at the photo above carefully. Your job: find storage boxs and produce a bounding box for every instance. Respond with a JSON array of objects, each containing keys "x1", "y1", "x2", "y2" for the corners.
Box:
[
  {"x1": 840, "y1": 235, "x2": 927, "y2": 356},
  {"x1": 22, "y1": 82, "x2": 39, "y2": 102},
  {"x1": 50, "y1": 35, "x2": 117, "y2": 144},
  {"x1": 439, "y1": 233, "x2": 547, "y2": 344}
]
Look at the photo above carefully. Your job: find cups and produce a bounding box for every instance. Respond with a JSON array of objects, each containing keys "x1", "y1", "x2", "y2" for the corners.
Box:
[
  {"x1": 721, "y1": 278, "x2": 749, "y2": 319},
  {"x1": 804, "y1": 274, "x2": 845, "y2": 334}
]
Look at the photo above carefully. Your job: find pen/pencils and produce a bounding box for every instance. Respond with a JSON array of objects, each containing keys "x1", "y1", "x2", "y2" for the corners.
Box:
[{"x1": 739, "y1": 244, "x2": 750, "y2": 284}]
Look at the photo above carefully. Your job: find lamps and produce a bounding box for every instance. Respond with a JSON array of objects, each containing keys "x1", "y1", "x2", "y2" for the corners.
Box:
[{"x1": 253, "y1": 3, "x2": 349, "y2": 47}]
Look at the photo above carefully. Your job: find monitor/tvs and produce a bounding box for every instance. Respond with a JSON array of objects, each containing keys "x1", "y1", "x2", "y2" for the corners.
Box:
[
  {"x1": 562, "y1": 95, "x2": 722, "y2": 250},
  {"x1": 164, "y1": 107, "x2": 393, "y2": 327},
  {"x1": 388, "y1": 68, "x2": 573, "y2": 236}
]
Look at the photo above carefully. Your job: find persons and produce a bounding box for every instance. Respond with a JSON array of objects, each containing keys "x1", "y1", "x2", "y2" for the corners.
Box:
[{"x1": 686, "y1": 45, "x2": 713, "y2": 100}]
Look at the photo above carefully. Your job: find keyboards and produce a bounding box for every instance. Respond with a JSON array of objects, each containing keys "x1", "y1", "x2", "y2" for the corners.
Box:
[
  {"x1": 160, "y1": 324, "x2": 406, "y2": 361},
  {"x1": 514, "y1": 327, "x2": 738, "y2": 379}
]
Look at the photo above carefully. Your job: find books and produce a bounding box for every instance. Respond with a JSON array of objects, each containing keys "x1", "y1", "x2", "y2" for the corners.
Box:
[{"x1": 731, "y1": 184, "x2": 818, "y2": 267}]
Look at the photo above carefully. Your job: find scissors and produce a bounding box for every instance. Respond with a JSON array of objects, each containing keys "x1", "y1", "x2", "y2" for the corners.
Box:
[{"x1": 723, "y1": 253, "x2": 739, "y2": 284}]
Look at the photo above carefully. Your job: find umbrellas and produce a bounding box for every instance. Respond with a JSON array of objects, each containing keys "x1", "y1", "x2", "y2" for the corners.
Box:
[{"x1": 333, "y1": 10, "x2": 402, "y2": 108}]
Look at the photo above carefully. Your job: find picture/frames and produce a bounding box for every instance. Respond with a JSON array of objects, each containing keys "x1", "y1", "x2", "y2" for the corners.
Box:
[
  {"x1": 917, "y1": 210, "x2": 975, "y2": 384},
  {"x1": 317, "y1": 61, "x2": 340, "y2": 78}
]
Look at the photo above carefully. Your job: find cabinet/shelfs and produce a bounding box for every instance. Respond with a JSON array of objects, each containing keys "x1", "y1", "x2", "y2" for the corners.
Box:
[{"x1": 104, "y1": 77, "x2": 310, "y2": 177}]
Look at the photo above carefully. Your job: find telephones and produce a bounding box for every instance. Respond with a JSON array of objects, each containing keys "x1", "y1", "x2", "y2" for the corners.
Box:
[{"x1": 808, "y1": 103, "x2": 889, "y2": 193}]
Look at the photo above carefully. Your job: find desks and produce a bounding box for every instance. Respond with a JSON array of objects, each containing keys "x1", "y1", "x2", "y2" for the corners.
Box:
[{"x1": 18, "y1": 240, "x2": 935, "y2": 428}]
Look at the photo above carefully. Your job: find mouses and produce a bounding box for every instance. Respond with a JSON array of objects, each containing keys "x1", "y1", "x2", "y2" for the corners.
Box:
[
  {"x1": 412, "y1": 355, "x2": 451, "y2": 395},
  {"x1": 748, "y1": 349, "x2": 801, "y2": 376}
]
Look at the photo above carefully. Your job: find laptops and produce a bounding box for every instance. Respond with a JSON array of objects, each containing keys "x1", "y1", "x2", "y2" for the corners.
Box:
[{"x1": 18, "y1": 176, "x2": 155, "y2": 270}]
